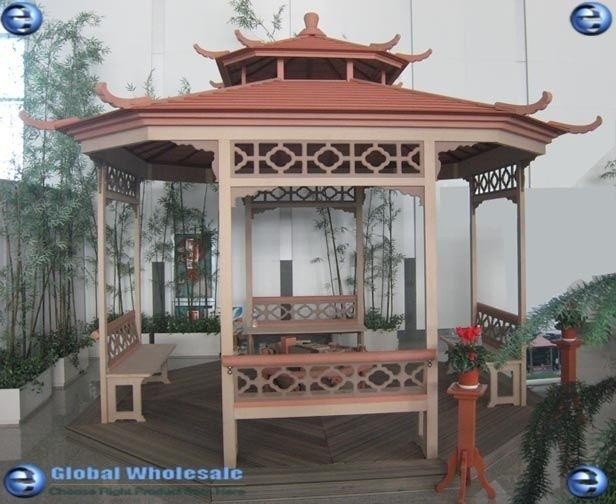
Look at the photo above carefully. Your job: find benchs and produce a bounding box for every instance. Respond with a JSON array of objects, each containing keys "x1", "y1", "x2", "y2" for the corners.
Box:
[{"x1": 439, "y1": 302, "x2": 527, "y2": 408}]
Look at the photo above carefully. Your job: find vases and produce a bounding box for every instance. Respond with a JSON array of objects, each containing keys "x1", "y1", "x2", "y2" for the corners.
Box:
[{"x1": 456, "y1": 368, "x2": 482, "y2": 390}]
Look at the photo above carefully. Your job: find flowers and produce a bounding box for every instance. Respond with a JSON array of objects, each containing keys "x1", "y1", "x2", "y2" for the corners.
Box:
[{"x1": 444, "y1": 326, "x2": 495, "y2": 374}]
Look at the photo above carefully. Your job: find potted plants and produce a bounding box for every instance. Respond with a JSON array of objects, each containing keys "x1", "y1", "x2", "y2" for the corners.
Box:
[{"x1": 0, "y1": 0, "x2": 407, "y2": 427}]
